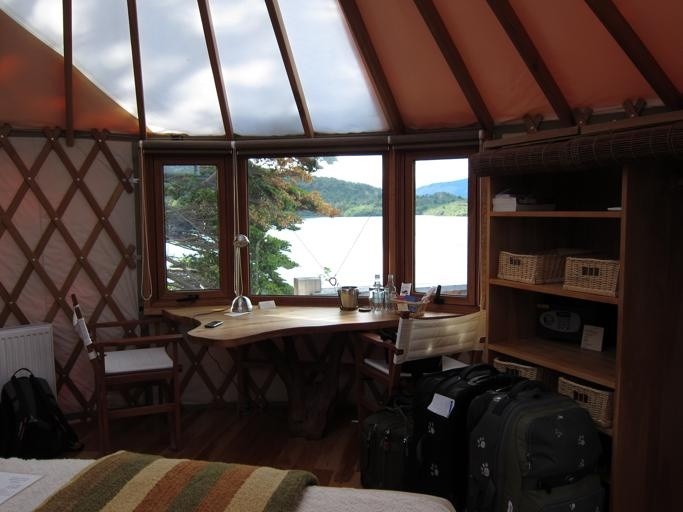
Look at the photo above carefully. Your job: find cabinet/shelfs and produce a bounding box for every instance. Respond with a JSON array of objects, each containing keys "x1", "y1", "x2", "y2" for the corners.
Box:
[{"x1": 480, "y1": 162, "x2": 683, "y2": 512}]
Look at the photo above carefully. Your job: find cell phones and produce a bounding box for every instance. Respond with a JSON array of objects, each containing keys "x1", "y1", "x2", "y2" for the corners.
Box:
[{"x1": 205, "y1": 321, "x2": 223, "y2": 328}]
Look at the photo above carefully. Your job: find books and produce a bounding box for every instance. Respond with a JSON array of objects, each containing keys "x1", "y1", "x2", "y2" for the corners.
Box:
[
  {"x1": 606, "y1": 207, "x2": 623, "y2": 211},
  {"x1": 492, "y1": 193, "x2": 555, "y2": 213}
]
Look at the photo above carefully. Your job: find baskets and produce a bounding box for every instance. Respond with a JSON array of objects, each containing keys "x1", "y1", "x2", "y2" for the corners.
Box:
[
  {"x1": 562, "y1": 256, "x2": 621, "y2": 296},
  {"x1": 494, "y1": 354, "x2": 544, "y2": 382},
  {"x1": 497, "y1": 248, "x2": 605, "y2": 285},
  {"x1": 558, "y1": 376, "x2": 613, "y2": 428}
]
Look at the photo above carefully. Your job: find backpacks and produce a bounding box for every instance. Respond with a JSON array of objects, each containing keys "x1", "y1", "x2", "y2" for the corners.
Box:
[{"x1": 2, "y1": 368, "x2": 85, "y2": 459}]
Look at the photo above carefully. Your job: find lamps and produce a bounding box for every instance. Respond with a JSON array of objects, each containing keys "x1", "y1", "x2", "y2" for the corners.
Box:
[{"x1": 230, "y1": 234, "x2": 253, "y2": 313}]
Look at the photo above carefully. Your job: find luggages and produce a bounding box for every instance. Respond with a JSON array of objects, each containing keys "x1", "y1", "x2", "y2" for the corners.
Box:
[
  {"x1": 417, "y1": 362, "x2": 526, "y2": 512},
  {"x1": 361, "y1": 403, "x2": 416, "y2": 490},
  {"x1": 466, "y1": 380, "x2": 607, "y2": 512}
]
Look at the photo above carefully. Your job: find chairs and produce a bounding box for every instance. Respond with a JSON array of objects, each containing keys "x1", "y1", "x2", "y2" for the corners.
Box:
[
  {"x1": 72, "y1": 293, "x2": 183, "y2": 452},
  {"x1": 357, "y1": 309, "x2": 486, "y2": 440}
]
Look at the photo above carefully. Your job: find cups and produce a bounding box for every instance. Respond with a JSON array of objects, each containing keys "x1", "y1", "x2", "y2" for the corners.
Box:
[{"x1": 339, "y1": 284, "x2": 359, "y2": 310}]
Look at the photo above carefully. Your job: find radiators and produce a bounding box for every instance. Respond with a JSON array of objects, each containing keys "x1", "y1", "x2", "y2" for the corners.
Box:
[{"x1": 0, "y1": 322, "x2": 57, "y2": 409}]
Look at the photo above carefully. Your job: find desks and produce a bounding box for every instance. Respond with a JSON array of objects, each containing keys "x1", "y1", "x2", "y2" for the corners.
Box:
[{"x1": 161, "y1": 303, "x2": 464, "y2": 442}]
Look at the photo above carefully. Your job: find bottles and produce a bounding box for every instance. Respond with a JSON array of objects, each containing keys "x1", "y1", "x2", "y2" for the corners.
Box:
[{"x1": 368, "y1": 273, "x2": 397, "y2": 313}]
